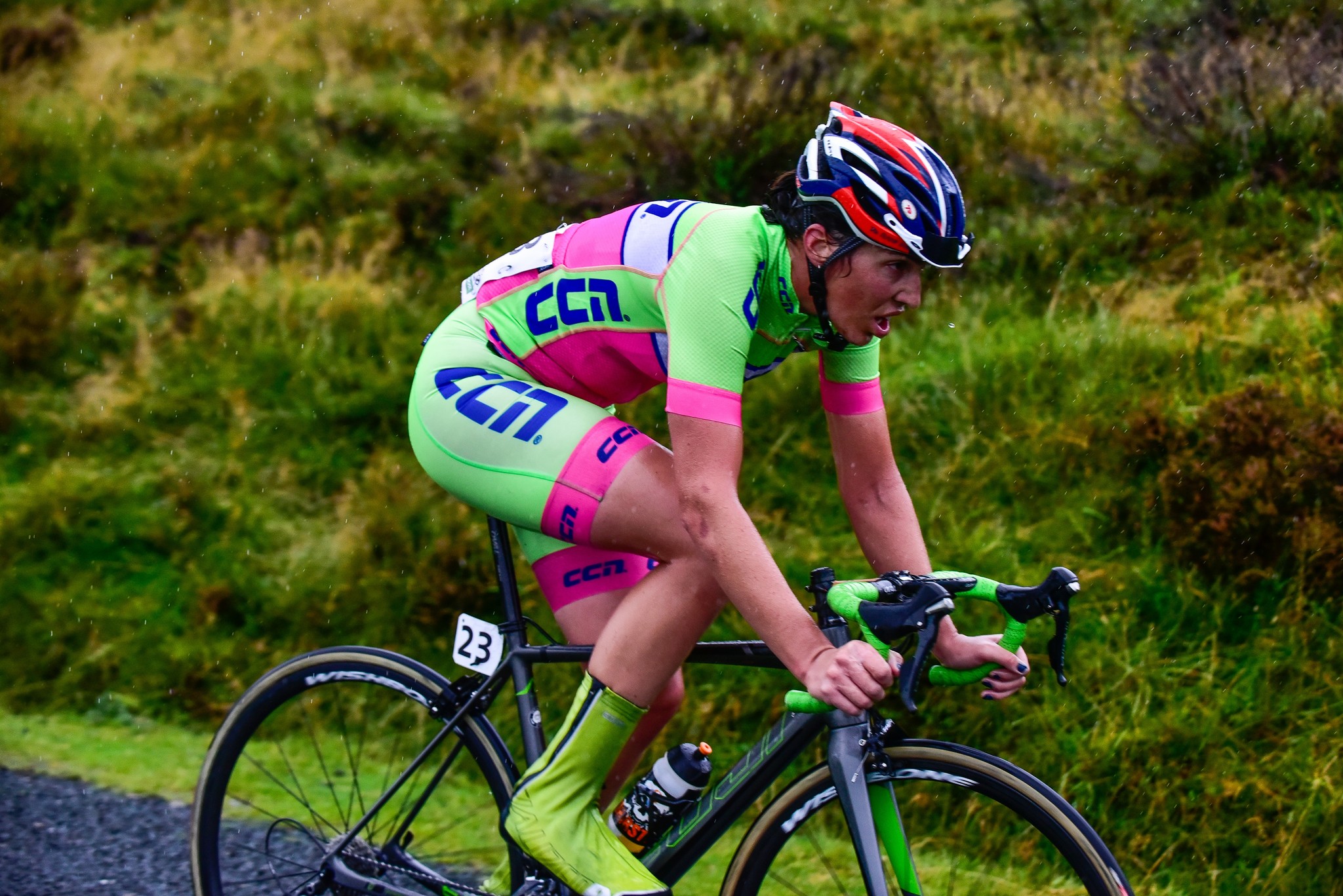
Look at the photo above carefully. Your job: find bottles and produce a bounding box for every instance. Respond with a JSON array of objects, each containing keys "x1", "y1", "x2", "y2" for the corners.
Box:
[{"x1": 608, "y1": 741, "x2": 712, "y2": 853}]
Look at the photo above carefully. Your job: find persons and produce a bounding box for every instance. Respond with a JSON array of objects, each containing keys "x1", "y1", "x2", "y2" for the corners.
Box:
[{"x1": 409, "y1": 102, "x2": 1031, "y2": 896}]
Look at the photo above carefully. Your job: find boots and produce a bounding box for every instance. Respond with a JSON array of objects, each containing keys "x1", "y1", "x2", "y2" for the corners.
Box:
[
  {"x1": 498, "y1": 669, "x2": 672, "y2": 896},
  {"x1": 479, "y1": 853, "x2": 512, "y2": 896}
]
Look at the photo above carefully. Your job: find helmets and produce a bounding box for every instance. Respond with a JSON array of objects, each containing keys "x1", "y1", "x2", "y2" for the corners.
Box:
[{"x1": 796, "y1": 101, "x2": 974, "y2": 268}]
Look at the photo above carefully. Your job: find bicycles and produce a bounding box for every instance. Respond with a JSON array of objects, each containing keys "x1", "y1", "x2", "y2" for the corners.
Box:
[{"x1": 193, "y1": 514, "x2": 1133, "y2": 895}]
